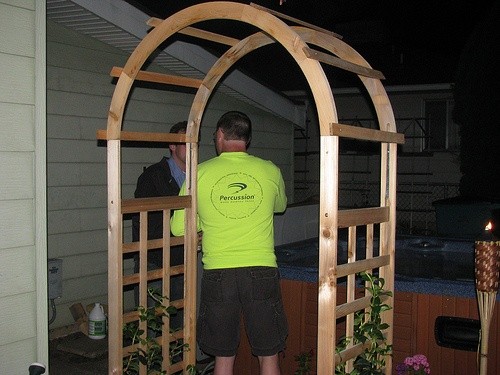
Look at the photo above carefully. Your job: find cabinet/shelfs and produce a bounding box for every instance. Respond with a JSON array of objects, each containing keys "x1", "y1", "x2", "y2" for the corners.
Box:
[{"x1": 232, "y1": 236, "x2": 500, "y2": 373}]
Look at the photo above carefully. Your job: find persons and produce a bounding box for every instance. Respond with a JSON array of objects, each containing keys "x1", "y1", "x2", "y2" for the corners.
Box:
[
  {"x1": 170, "y1": 112, "x2": 291, "y2": 375},
  {"x1": 130, "y1": 117, "x2": 206, "y2": 368}
]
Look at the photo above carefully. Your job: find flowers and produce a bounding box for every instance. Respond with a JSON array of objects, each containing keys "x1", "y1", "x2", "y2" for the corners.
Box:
[{"x1": 395, "y1": 353, "x2": 432, "y2": 375}]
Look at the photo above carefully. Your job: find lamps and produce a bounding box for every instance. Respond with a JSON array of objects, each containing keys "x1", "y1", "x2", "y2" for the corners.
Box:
[{"x1": 475, "y1": 222, "x2": 500, "y2": 375}]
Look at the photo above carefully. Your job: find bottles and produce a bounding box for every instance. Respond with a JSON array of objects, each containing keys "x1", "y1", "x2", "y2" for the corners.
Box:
[{"x1": 88, "y1": 303, "x2": 106, "y2": 340}]
